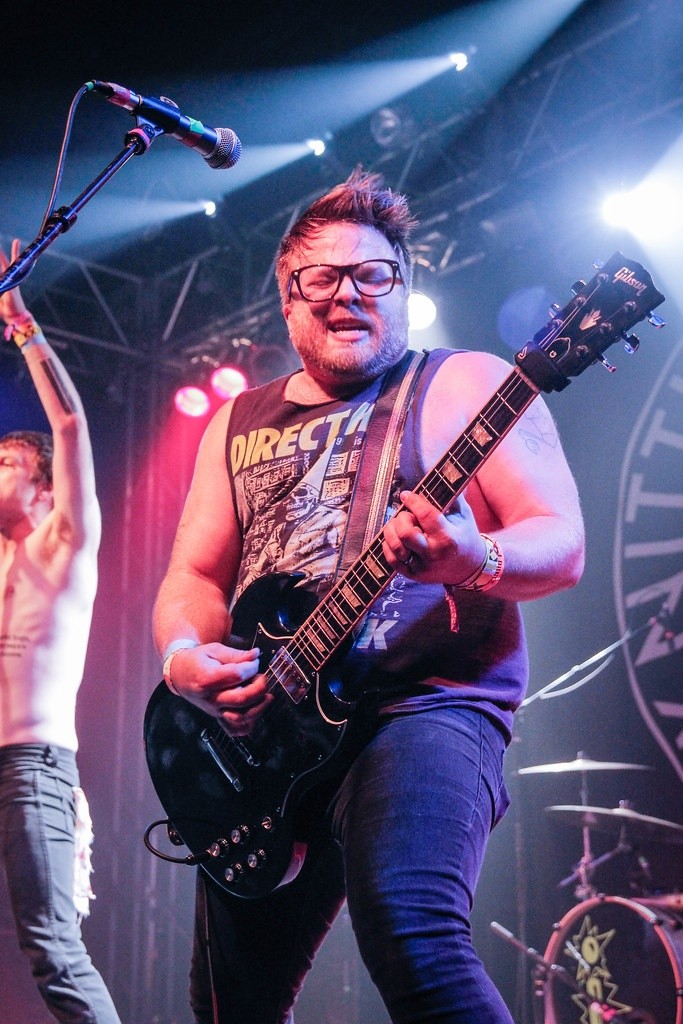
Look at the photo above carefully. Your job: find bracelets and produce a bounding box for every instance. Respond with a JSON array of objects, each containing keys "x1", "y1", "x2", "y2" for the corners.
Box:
[
  {"x1": 439, "y1": 533, "x2": 505, "y2": 634},
  {"x1": 162, "y1": 639, "x2": 199, "y2": 697},
  {"x1": 5, "y1": 311, "x2": 46, "y2": 352}
]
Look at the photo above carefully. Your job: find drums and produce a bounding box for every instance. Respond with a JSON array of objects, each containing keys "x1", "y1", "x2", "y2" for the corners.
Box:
[{"x1": 533, "y1": 892, "x2": 683, "y2": 1024}]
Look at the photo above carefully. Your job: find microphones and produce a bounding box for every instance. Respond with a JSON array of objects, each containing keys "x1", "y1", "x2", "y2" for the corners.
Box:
[
  {"x1": 662, "y1": 610, "x2": 675, "y2": 652},
  {"x1": 93, "y1": 80, "x2": 242, "y2": 170}
]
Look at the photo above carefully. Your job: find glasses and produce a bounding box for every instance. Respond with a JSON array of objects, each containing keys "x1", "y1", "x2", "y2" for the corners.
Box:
[{"x1": 287, "y1": 258, "x2": 406, "y2": 301}]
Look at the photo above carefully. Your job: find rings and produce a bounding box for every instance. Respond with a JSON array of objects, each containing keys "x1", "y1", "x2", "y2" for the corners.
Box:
[
  {"x1": 218, "y1": 710, "x2": 225, "y2": 720},
  {"x1": 402, "y1": 551, "x2": 414, "y2": 565}
]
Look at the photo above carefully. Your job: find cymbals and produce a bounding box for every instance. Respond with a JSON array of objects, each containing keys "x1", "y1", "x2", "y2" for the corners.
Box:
[
  {"x1": 516, "y1": 759, "x2": 653, "y2": 774},
  {"x1": 546, "y1": 804, "x2": 683, "y2": 845}
]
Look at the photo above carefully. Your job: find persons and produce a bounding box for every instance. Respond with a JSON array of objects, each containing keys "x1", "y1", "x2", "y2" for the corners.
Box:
[
  {"x1": 0, "y1": 238, "x2": 126, "y2": 1024},
  {"x1": 146, "y1": 153, "x2": 584, "y2": 1015}
]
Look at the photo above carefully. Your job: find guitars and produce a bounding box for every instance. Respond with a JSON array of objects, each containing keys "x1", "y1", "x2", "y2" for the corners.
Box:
[{"x1": 141, "y1": 249, "x2": 668, "y2": 903}]
